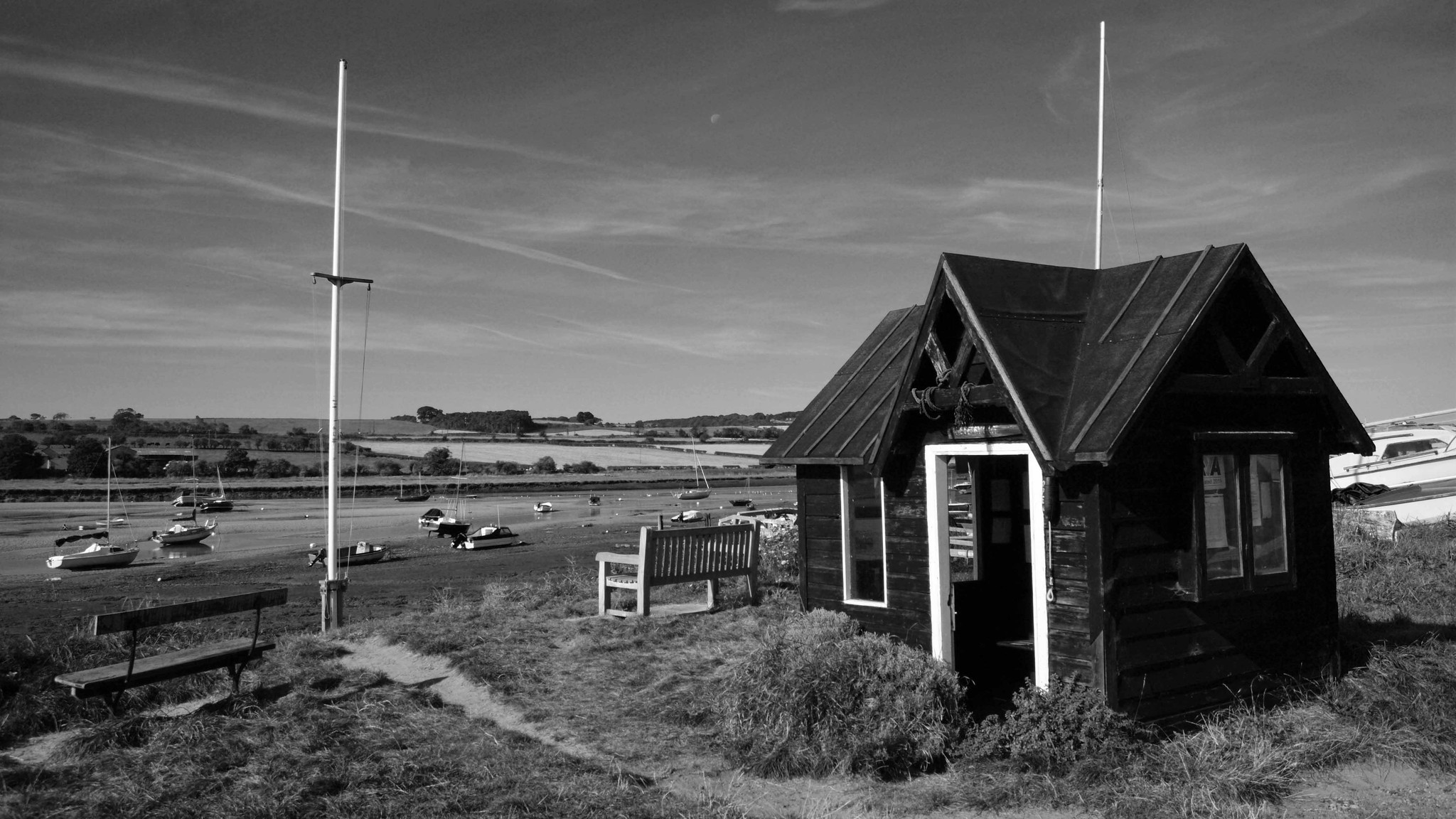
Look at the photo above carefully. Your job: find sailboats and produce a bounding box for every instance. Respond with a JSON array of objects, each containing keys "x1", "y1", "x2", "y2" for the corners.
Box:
[
  {"x1": 151, "y1": 435, "x2": 217, "y2": 544},
  {"x1": 200, "y1": 465, "x2": 234, "y2": 513},
  {"x1": 729, "y1": 472, "x2": 753, "y2": 506},
  {"x1": 418, "y1": 440, "x2": 471, "y2": 534},
  {"x1": 670, "y1": 435, "x2": 712, "y2": 499},
  {"x1": 45, "y1": 438, "x2": 139, "y2": 569},
  {"x1": 394, "y1": 471, "x2": 431, "y2": 501}
]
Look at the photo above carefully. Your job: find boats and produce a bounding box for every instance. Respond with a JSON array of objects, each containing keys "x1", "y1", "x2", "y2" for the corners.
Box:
[
  {"x1": 671, "y1": 510, "x2": 702, "y2": 522},
  {"x1": 466, "y1": 495, "x2": 477, "y2": 498},
  {"x1": 95, "y1": 519, "x2": 124, "y2": 526},
  {"x1": 1347, "y1": 476, "x2": 1456, "y2": 528},
  {"x1": 588, "y1": 496, "x2": 601, "y2": 506},
  {"x1": 172, "y1": 479, "x2": 213, "y2": 507},
  {"x1": 307, "y1": 541, "x2": 391, "y2": 568},
  {"x1": 450, "y1": 505, "x2": 519, "y2": 549},
  {"x1": 1329, "y1": 420, "x2": 1456, "y2": 492},
  {"x1": 534, "y1": 501, "x2": 553, "y2": 512}
]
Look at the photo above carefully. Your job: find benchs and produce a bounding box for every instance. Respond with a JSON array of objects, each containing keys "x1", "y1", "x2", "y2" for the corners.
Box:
[
  {"x1": 594, "y1": 518, "x2": 762, "y2": 618},
  {"x1": 55, "y1": 588, "x2": 288, "y2": 720}
]
